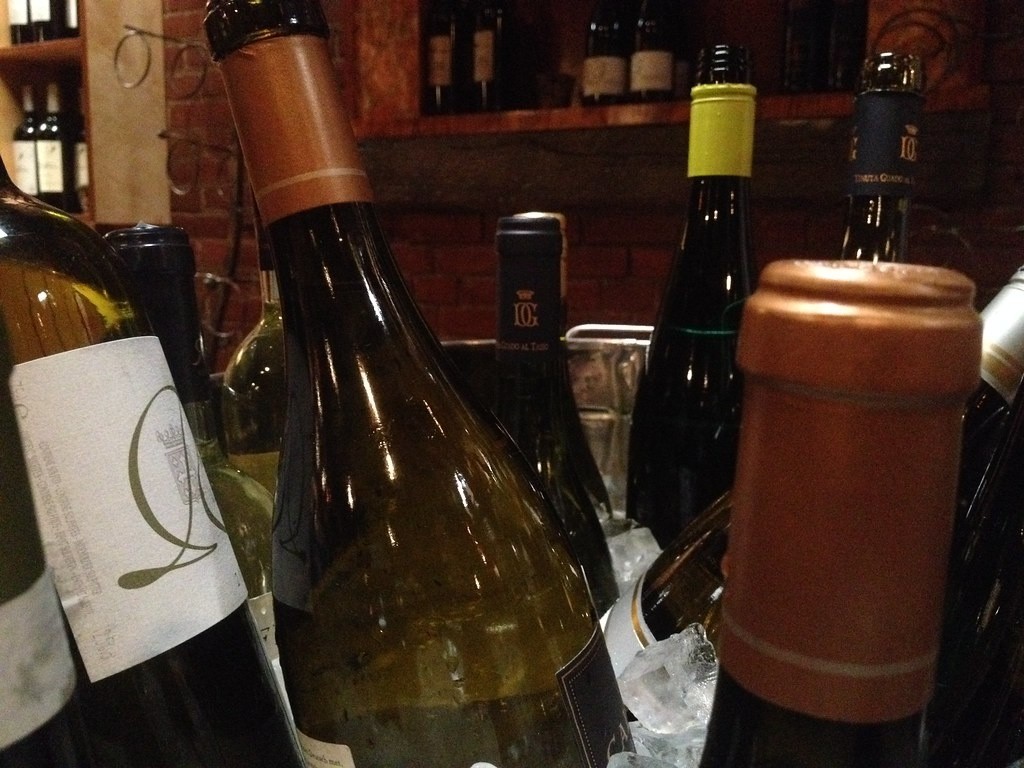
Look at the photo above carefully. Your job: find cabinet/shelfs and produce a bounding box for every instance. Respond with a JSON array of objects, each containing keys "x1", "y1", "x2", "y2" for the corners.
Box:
[
  {"x1": 1, "y1": 0, "x2": 96, "y2": 220},
  {"x1": 348, "y1": 1, "x2": 989, "y2": 138}
]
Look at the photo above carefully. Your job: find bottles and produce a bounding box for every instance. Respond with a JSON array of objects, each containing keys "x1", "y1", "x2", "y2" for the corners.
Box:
[
  {"x1": 771, "y1": 0, "x2": 869, "y2": 93},
  {"x1": 579, "y1": 0, "x2": 682, "y2": 107},
  {"x1": 0, "y1": 152, "x2": 309, "y2": 768},
  {"x1": 420, "y1": 0, "x2": 508, "y2": 114},
  {"x1": 696, "y1": 254, "x2": 984, "y2": 768},
  {"x1": 10, "y1": 82, "x2": 89, "y2": 215},
  {"x1": 199, "y1": 0, "x2": 639, "y2": 768},
  {"x1": 6, "y1": 0, "x2": 79, "y2": 44},
  {"x1": 835, "y1": 48, "x2": 931, "y2": 285},
  {"x1": 595, "y1": 264, "x2": 1024, "y2": 739},
  {"x1": 626, "y1": 40, "x2": 762, "y2": 534},
  {"x1": 485, "y1": 211, "x2": 615, "y2": 546}
]
